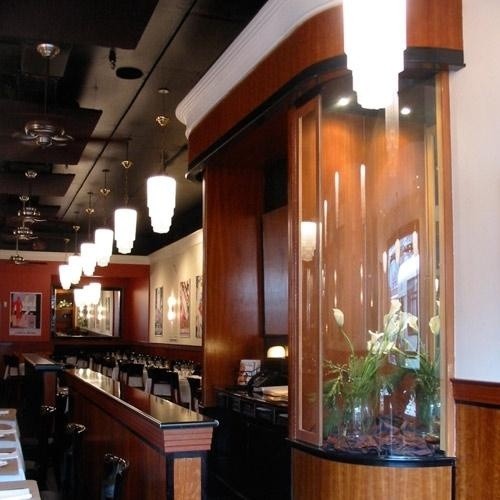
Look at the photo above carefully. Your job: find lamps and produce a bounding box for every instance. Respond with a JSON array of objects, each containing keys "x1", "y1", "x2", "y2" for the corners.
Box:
[
  {"x1": 246, "y1": 344, "x2": 288, "y2": 398},
  {"x1": 57, "y1": 88, "x2": 178, "y2": 291}
]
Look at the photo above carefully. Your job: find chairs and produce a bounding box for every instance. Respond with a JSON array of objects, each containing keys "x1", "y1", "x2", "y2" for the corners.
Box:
[{"x1": 0, "y1": 348, "x2": 221, "y2": 500}]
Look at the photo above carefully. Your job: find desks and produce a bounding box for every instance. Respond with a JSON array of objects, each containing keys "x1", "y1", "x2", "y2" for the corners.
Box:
[{"x1": 214, "y1": 386, "x2": 289, "y2": 430}]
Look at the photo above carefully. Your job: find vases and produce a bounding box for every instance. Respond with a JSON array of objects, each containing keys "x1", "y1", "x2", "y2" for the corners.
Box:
[
  {"x1": 415, "y1": 385, "x2": 443, "y2": 431},
  {"x1": 340, "y1": 389, "x2": 379, "y2": 440}
]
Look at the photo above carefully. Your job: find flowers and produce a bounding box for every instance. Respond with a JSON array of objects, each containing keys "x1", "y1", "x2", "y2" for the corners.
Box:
[
  {"x1": 319, "y1": 296, "x2": 404, "y2": 436},
  {"x1": 393, "y1": 300, "x2": 443, "y2": 425}
]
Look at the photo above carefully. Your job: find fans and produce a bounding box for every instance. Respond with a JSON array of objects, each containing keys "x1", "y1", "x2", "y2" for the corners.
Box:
[{"x1": 0, "y1": 38, "x2": 137, "y2": 266}]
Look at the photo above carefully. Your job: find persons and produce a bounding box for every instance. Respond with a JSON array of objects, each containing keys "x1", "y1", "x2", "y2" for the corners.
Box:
[{"x1": 13, "y1": 296, "x2": 23, "y2": 321}]
